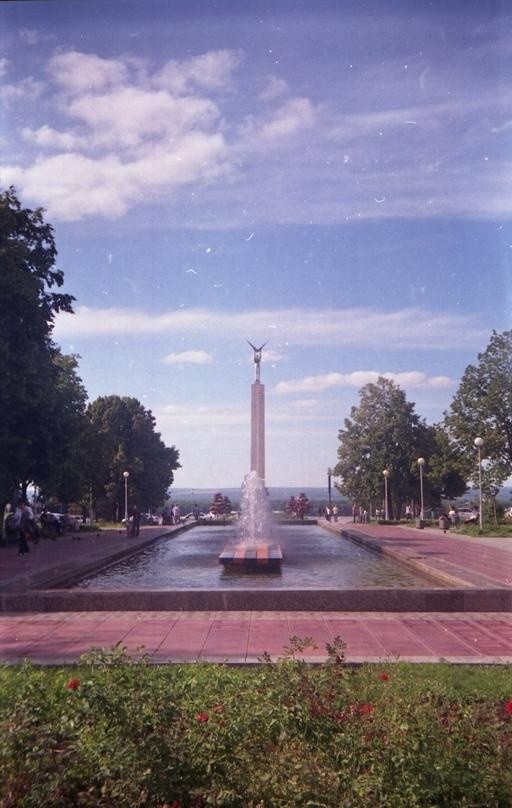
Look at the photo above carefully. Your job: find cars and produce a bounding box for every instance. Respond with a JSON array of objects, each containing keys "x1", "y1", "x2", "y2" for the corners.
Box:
[
  {"x1": 180, "y1": 512, "x2": 212, "y2": 524},
  {"x1": 448, "y1": 508, "x2": 480, "y2": 523},
  {"x1": 48, "y1": 513, "x2": 90, "y2": 523},
  {"x1": 121, "y1": 513, "x2": 160, "y2": 525}
]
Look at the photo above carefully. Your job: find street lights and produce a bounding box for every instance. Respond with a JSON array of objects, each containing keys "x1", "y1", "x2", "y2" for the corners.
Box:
[
  {"x1": 327, "y1": 467, "x2": 332, "y2": 503},
  {"x1": 121, "y1": 469, "x2": 130, "y2": 524},
  {"x1": 474, "y1": 437, "x2": 485, "y2": 530},
  {"x1": 382, "y1": 469, "x2": 394, "y2": 520},
  {"x1": 417, "y1": 457, "x2": 426, "y2": 520}
]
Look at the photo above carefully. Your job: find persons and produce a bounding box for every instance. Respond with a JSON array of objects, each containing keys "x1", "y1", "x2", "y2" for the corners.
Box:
[
  {"x1": 122, "y1": 501, "x2": 142, "y2": 537},
  {"x1": 10, "y1": 500, "x2": 34, "y2": 559},
  {"x1": 41, "y1": 508, "x2": 49, "y2": 520},
  {"x1": 64, "y1": 511, "x2": 87, "y2": 539},
  {"x1": 406, "y1": 504, "x2": 411, "y2": 521},
  {"x1": 193, "y1": 503, "x2": 200, "y2": 520},
  {"x1": 351, "y1": 503, "x2": 369, "y2": 523},
  {"x1": 449, "y1": 506, "x2": 458, "y2": 528},
  {"x1": 325, "y1": 504, "x2": 340, "y2": 522},
  {"x1": 170, "y1": 503, "x2": 180, "y2": 525}
]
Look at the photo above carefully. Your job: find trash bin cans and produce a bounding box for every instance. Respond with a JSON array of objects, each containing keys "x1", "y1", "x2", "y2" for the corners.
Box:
[
  {"x1": 416, "y1": 519, "x2": 424, "y2": 529},
  {"x1": 431, "y1": 510, "x2": 439, "y2": 519},
  {"x1": 439, "y1": 516, "x2": 450, "y2": 535},
  {"x1": 365, "y1": 512, "x2": 369, "y2": 524}
]
